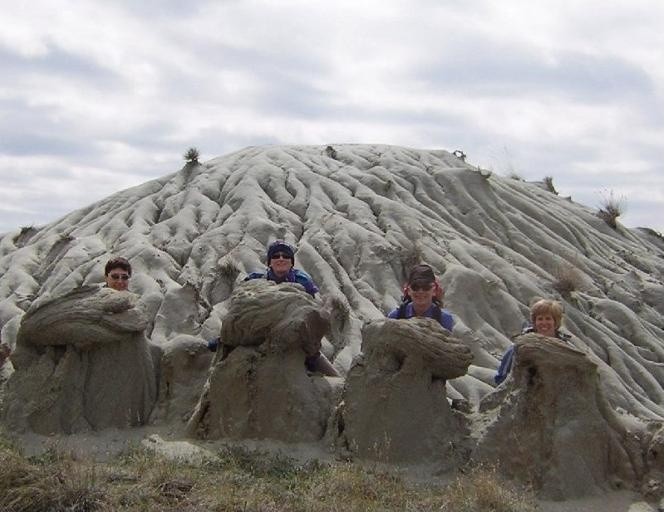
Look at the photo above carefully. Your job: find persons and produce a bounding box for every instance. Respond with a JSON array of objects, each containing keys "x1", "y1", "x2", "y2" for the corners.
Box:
[
  {"x1": 206, "y1": 241, "x2": 339, "y2": 379},
  {"x1": 389, "y1": 262, "x2": 453, "y2": 333},
  {"x1": 103, "y1": 257, "x2": 131, "y2": 292},
  {"x1": 493, "y1": 300, "x2": 573, "y2": 386}
]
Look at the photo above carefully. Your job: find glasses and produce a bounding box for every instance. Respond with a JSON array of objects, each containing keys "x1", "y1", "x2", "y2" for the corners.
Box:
[
  {"x1": 271, "y1": 252, "x2": 291, "y2": 259},
  {"x1": 107, "y1": 273, "x2": 130, "y2": 281},
  {"x1": 407, "y1": 283, "x2": 436, "y2": 291}
]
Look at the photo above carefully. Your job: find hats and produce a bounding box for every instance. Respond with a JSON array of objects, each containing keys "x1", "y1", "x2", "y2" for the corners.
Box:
[
  {"x1": 267, "y1": 239, "x2": 294, "y2": 266},
  {"x1": 409, "y1": 265, "x2": 435, "y2": 282}
]
